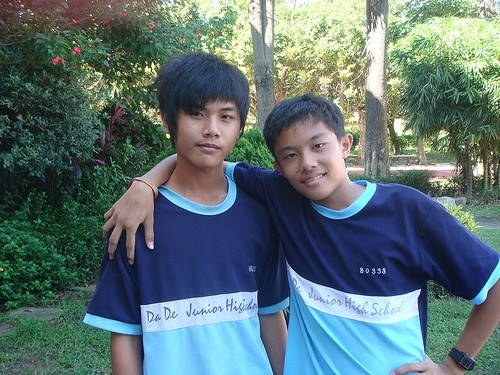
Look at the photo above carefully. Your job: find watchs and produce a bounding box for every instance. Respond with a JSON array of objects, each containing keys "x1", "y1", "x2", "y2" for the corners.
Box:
[{"x1": 448, "y1": 347, "x2": 477, "y2": 371}]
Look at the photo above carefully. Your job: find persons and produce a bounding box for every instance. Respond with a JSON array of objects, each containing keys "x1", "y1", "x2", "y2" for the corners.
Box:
[
  {"x1": 83, "y1": 51, "x2": 291, "y2": 375},
  {"x1": 102, "y1": 92, "x2": 500, "y2": 375}
]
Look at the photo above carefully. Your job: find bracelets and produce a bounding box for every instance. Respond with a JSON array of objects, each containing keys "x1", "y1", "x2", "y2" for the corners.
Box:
[{"x1": 128, "y1": 176, "x2": 159, "y2": 199}]
